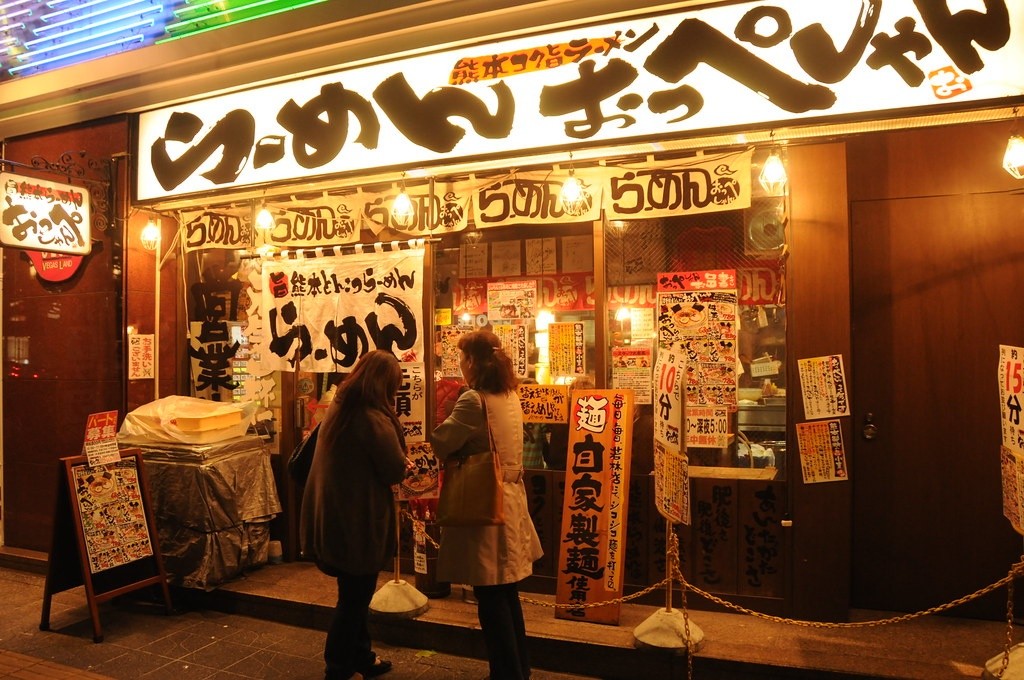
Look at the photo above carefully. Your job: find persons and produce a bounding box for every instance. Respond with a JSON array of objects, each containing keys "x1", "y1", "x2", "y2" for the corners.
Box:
[
  {"x1": 299, "y1": 349, "x2": 416, "y2": 680},
  {"x1": 433, "y1": 350, "x2": 654, "y2": 476},
  {"x1": 428, "y1": 331, "x2": 545, "y2": 680}
]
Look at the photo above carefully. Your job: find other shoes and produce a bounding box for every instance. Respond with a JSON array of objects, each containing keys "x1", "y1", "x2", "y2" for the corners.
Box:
[{"x1": 367, "y1": 658, "x2": 392, "y2": 676}]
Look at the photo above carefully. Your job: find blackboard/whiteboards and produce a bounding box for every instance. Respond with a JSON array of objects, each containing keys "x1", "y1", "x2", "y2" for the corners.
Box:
[{"x1": 65, "y1": 447, "x2": 165, "y2": 604}]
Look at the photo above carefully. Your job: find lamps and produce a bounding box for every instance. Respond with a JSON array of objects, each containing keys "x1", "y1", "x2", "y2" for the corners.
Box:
[
  {"x1": 254, "y1": 190, "x2": 277, "y2": 230},
  {"x1": 1003, "y1": 106, "x2": 1024, "y2": 180},
  {"x1": 141, "y1": 215, "x2": 161, "y2": 250},
  {"x1": 757, "y1": 129, "x2": 788, "y2": 195},
  {"x1": 390, "y1": 172, "x2": 414, "y2": 226},
  {"x1": 559, "y1": 153, "x2": 584, "y2": 215}
]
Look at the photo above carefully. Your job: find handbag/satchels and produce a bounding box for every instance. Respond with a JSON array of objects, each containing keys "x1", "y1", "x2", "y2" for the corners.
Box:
[
  {"x1": 287, "y1": 421, "x2": 322, "y2": 489},
  {"x1": 437, "y1": 388, "x2": 505, "y2": 527}
]
